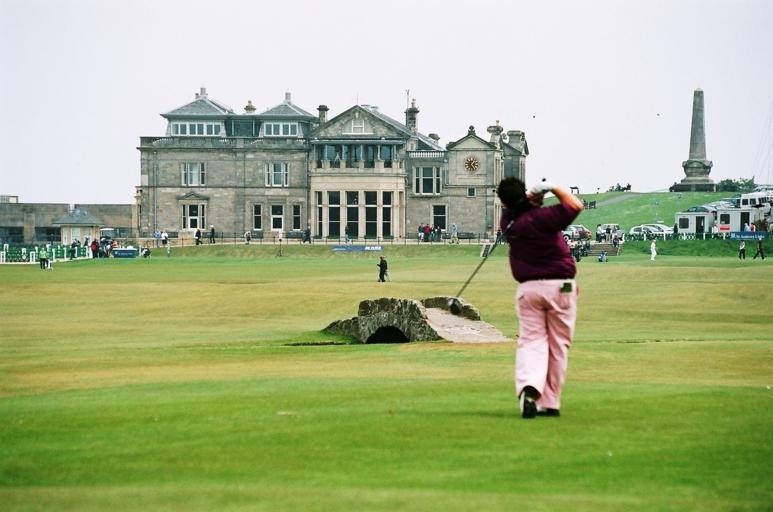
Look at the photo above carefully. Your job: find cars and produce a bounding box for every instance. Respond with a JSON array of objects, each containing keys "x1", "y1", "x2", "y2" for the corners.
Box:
[
  {"x1": 682, "y1": 197, "x2": 740, "y2": 219},
  {"x1": 562, "y1": 224, "x2": 674, "y2": 242}
]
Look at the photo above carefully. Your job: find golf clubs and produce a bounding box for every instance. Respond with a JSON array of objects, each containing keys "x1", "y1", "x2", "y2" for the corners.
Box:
[{"x1": 448, "y1": 177, "x2": 546, "y2": 316}]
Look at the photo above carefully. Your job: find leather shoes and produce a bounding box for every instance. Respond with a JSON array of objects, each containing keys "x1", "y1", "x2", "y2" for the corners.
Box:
[{"x1": 518, "y1": 386, "x2": 561, "y2": 419}]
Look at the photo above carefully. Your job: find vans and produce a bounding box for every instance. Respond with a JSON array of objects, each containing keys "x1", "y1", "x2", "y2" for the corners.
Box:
[{"x1": 740, "y1": 192, "x2": 771, "y2": 215}]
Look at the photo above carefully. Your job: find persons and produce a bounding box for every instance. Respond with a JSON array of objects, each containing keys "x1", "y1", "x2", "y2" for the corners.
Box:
[
  {"x1": 596, "y1": 224, "x2": 624, "y2": 252},
  {"x1": 753, "y1": 236, "x2": 765, "y2": 260},
  {"x1": 154, "y1": 229, "x2": 169, "y2": 248},
  {"x1": 599, "y1": 248, "x2": 607, "y2": 261},
  {"x1": 345, "y1": 225, "x2": 352, "y2": 243},
  {"x1": 615, "y1": 183, "x2": 631, "y2": 191},
  {"x1": 569, "y1": 229, "x2": 590, "y2": 262},
  {"x1": 497, "y1": 177, "x2": 585, "y2": 418},
  {"x1": 418, "y1": 223, "x2": 441, "y2": 242},
  {"x1": 210, "y1": 227, "x2": 215, "y2": 243},
  {"x1": 195, "y1": 228, "x2": 202, "y2": 245},
  {"x1": 377, "y1": 255, "x2": 387, "y2": 282},
  {"x1": 304, "y1": 226, "x2": 311, "y2": 244},
  {"x1": 672, "y1": 223, "x2": 679, "y2": 241},
  {"x1": 245, "y1": 231, "x2": 253, "y2": 242},
  {"x1": 651, "y1": 240, "x2": 656, "y2": 260},
  {"x1": 39, "y1": 248, "x2": 48, "y2": 271},
  {"x1": 739, "y1": 240, "x2": 745, "y2": 259},
  {"x1": 497, "y1": 230, "x2": 501, "y2": 244},
  {"x1": 70, "y1": 236, "x2": 119, "y2": 260},
  {"x1": 744, "y1": 223, "x2": 755, "y2": 232},
  {"x1": 449, "y1": 223, "x2": 459, "y2": 244}
]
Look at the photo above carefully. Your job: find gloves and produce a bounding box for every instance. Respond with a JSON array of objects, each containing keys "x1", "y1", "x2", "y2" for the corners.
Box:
[{"x1": 531, "y1": 180, "x2": 553, "y2": 195}]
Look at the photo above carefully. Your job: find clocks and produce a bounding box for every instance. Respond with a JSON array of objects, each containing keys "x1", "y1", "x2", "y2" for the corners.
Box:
[{"x1": 463, "y1": 154, "x2": 480, "y2": 173}]
